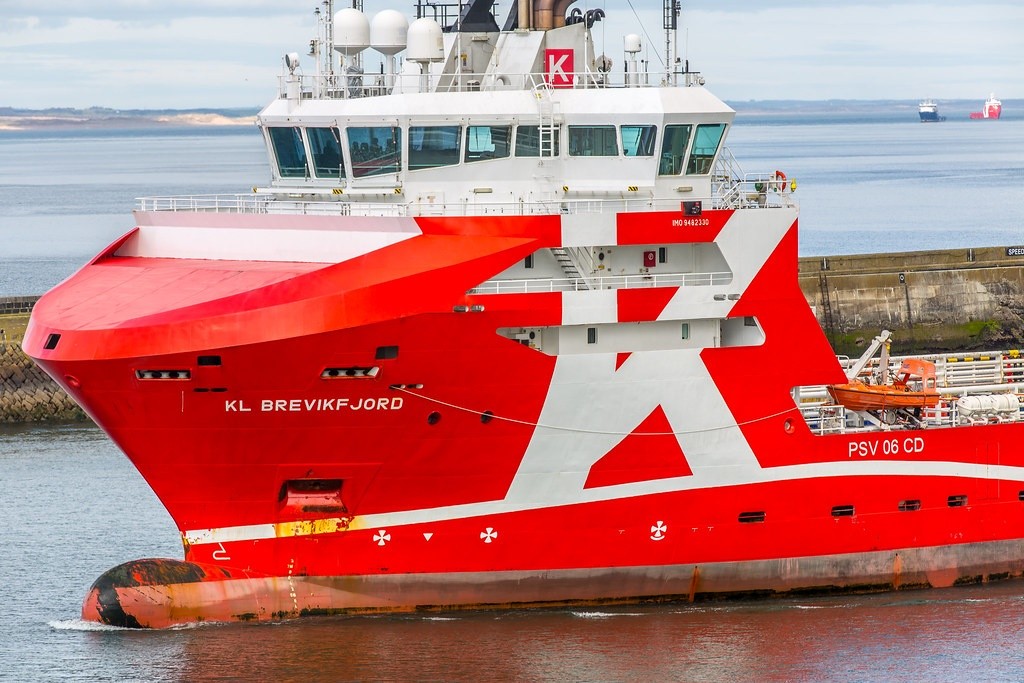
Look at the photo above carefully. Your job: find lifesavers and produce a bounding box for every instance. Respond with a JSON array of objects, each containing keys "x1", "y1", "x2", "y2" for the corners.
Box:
[{"x1": 775, "y1": 170, "x2": 787, "y2": 191}]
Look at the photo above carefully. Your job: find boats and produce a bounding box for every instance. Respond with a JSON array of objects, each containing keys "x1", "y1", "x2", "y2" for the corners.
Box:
[
  {"x1": 21, "y1": 0, "x2": 1024, "y2": 629},
  {"x1": 917, "y1": 98, "x2": 947, "y2": 122},
  {"x1": 969, "y1": 93, "x2": 1001, "y2": 119}
]
